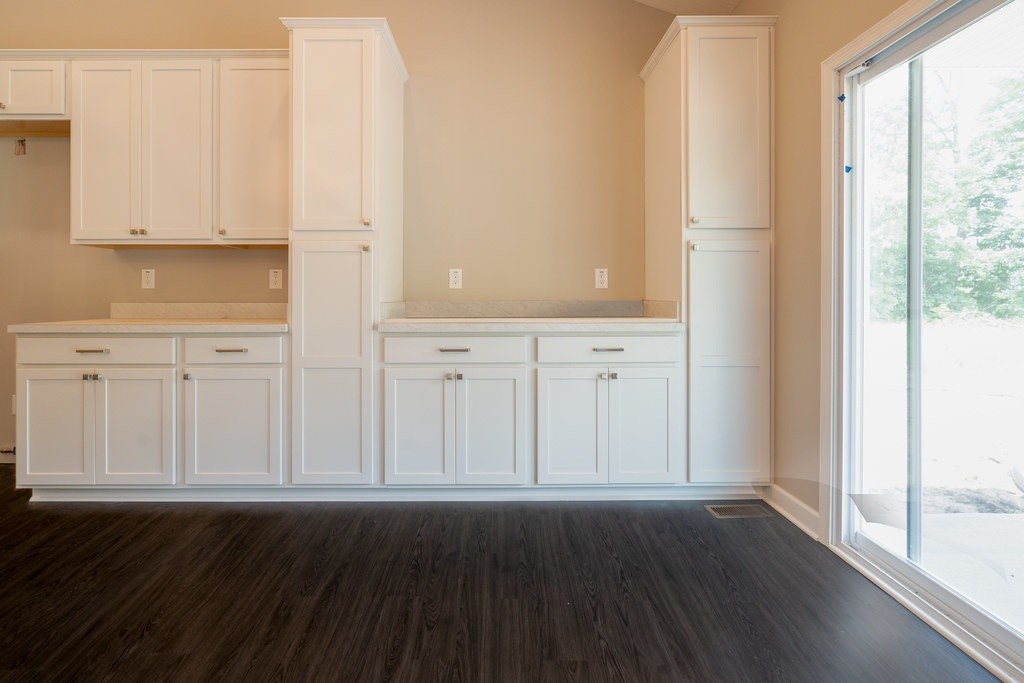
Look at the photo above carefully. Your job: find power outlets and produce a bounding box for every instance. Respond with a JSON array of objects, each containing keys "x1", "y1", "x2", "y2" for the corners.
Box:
[
  {"x1": 447, "y1": 268, "x2": 462, "y2": 289},
  {"x1": 141, "y1": 268, "x2": 154, "y2": 288},
  {"x1": 594, "y1": 268, "x2": 608, "y2": 288},
  {"x1": 268, "y1": 268, "x2": 282, "y2": 289}
]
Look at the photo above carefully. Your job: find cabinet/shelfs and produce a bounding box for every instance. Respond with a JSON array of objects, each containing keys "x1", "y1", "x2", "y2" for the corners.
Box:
[
  {"x1": 0, "y1": 48, "x2": 70, "y2": 135},
  {"x1": 635, "y1": 13, "x2": 779, "y2": 502},
  {"x1": 278, "y1": 16, "x2": 408, "y2": 501},
  {"x1": 530, "y1": 332, "x2": 686, "y2": 500},
  {"x1": 212, "y1": 48, "x2": 289, "y2": 244},
  {"x1": 70, "y1": 48, "x2": 213, "y2": 247},
  {"x1": 14, "y1": 332, "x2": 182, "y2": 501},
  {"x1": 381, "y1": 333, "x2": 532, "y2": 500},
  {"x1": 179, "y1": 332, "x2": 284, "y2": 501}
]
